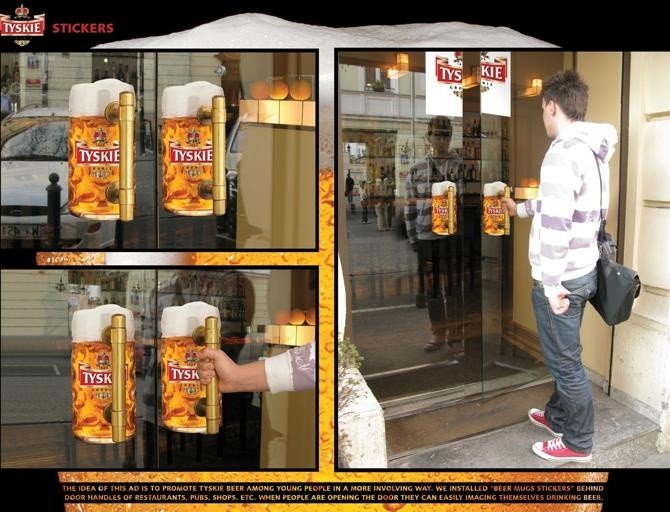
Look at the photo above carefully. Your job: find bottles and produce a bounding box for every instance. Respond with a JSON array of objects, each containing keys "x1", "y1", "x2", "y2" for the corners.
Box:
[
  {"x1": 0, "y1": 61, "x2": 19, "y2": 84},
  {"x1": 67, "y1": 268, "x2": 245, "y2": 377},
  {"x1": 368, "y1": 117, "x2": 509, "y2": 196},
  {"x1": 94, "y1": 62, "x2": 143, "y2": 92}
]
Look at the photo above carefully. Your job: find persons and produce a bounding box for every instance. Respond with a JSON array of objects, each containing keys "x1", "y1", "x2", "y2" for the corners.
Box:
[
  {"x1": 404, "y1": 115, "x2": 466, "y2": 363},
  {"x1": 145, "y1": 265, "x2": 257, "y2": 473},
  {"x1": 500, "y1": 70, "x2": 619, "y2": 464},
  {"x1": 346, "y1": 173, "x2": 395, "y2": 232},
  {"x1": 197, "y1": 342, "x2": 316, "y2": 394}
]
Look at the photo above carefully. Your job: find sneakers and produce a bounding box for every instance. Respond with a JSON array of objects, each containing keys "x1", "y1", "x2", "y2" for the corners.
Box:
[
  {"x1": 531, "y1": 437, "x2": 593, "y2": 464},
  {"x1": 425, "y1": 336, "x2": 445, "y2": 351},
  {"x1": 528, "y1": 406, "x2": 565, "y2": 438},
  {"x1": 448, "y1": 341, "x2": 465, "y2": 359}
]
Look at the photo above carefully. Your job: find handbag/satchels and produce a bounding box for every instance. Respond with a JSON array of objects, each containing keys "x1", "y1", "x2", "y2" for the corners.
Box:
[{"x1": 588, "y1": 255, "x2": 641, "y2": 326}]
